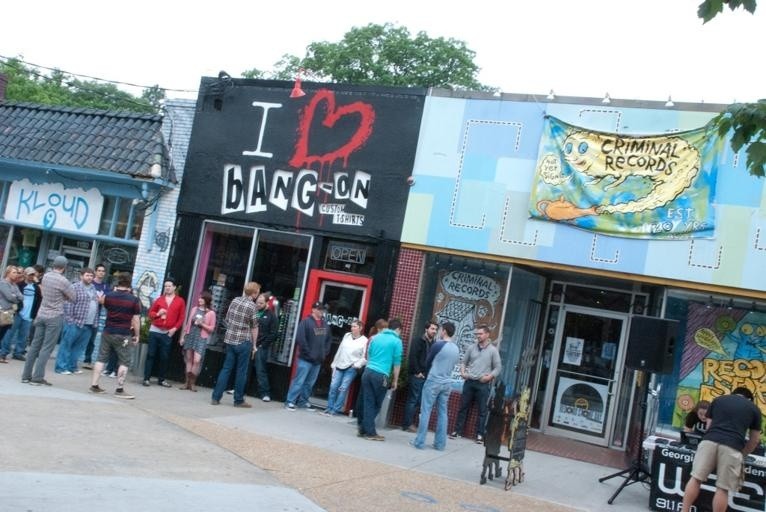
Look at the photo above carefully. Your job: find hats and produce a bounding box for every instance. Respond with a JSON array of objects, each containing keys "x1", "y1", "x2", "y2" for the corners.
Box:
[
  {"x1": 311, "y1": 302, "x2": 327, "y2": 309},
  {"x1": 23, "y1": 267, "x2": 36, "y2": 276}
]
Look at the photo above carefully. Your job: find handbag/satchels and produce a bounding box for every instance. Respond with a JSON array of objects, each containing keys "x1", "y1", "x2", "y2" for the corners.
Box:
[{"x1": 0, "y1": 309, "x2": 16, "y2": 326}]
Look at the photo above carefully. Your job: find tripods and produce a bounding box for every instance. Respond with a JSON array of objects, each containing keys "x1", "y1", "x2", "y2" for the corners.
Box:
[{"x1": 598, "y1": 370, "x2": 653, "y2": 505}]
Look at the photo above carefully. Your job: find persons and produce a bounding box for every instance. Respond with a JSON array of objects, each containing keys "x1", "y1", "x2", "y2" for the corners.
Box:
[
  {"x1": 450, "y1": 324, "x2": 502, "y2": 444},
  {"x1": 284, "y1": 300, "x2": 458, "y2": 447},
  {"x1": 0, "y1": 254, "x2": 142, "y2": 399},
  {"x1": 684, "y1": 400, "x2": 709, "y2": 433},
  {"x1": 143, "y1": 278, "x2": 278, "y2": 408},
  {"x1": 677, "y1": 386, "x2": 762, "y2": 512}
]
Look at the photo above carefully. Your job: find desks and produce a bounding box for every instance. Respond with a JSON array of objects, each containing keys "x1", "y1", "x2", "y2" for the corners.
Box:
[{"x1": 642, "y1": 436, "x2": 766, "y2": 512}]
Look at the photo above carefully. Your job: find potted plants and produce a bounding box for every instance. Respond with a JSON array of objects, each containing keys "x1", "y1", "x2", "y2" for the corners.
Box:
[{"x1": 132, "y1": 314, "x2": 152, "y2": 377}]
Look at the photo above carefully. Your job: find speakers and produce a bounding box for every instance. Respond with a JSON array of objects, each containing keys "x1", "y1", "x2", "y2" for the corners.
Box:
[{"x1": 624, "y1": 315, "x2": 680, "y2": 376}]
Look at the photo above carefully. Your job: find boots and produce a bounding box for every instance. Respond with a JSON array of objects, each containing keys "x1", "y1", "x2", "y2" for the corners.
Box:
[{"x1": 179, "y1": 372, "x2": 198, "y2": 392}]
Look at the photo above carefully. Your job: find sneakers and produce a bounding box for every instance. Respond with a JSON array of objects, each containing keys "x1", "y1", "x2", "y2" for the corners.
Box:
[
  {"x1": 233, "y1": 401, "x2": 251, "y2": 408},
  {"x1": 403, "y1": 424, "x2": 417, "y2": 432},
  {"x1": 101, "y1": 370, "x2": 117, "y2": 378},
  {"x1": 0, "y1": 353, "x2": 25, "y2": 362},
  {"x1": 356, "y1": 433, "x2": 384, "y2": 440},
  {"x1": 262, "y1": 395, "x2": 271, "y2": 402},
  {"x1": 21, "y1": 378, "x2": 53, "y2": 386},
  {"x1": 59, "y1": 362, "x2": 93, "y2": 375},
  {"x1": 211, "y1": 398, "x2": 219, "y2": 405},
  {"x1": 476, "y1": 434, "x2": 483, "y2": 444},
  {"x1": 142, "y1": 380, "x2": 150, "y2": 386},
  {"x1": 88, "y1": 386, "x2": 107, "y2": 394},
  {"x1": 158, "y1": 380, "x2": 171, "y2": 387},
  {"x1": 285, "y1": 400, "x2": 336, "y2": 414},
  {"x1": 449, "y1": 432, "x2": 461, "y2": 440},
  {"x1": 113, "y1": 390, "x2": 135, "y2": 399},
  {"x1": 227, "y1": 389, "x2": 235, "y2": 394}
]
